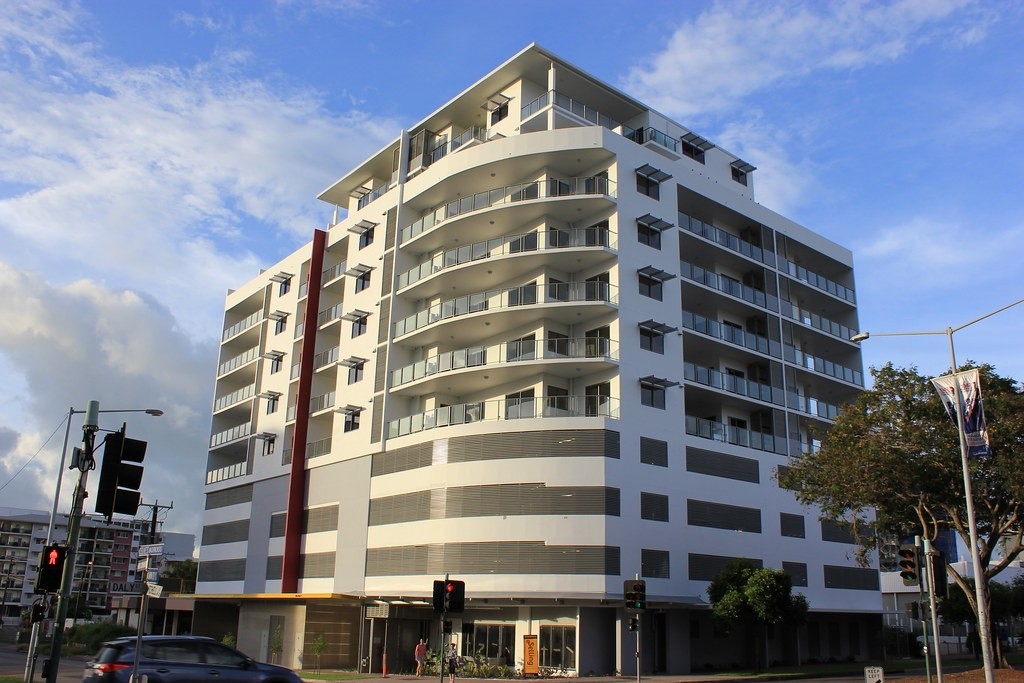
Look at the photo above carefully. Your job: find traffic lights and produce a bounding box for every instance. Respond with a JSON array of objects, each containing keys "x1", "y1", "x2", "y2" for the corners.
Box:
[
  {"x1": 905, "y1": 602, "x2": 920, "y2": 621},
  {"x1": 95, "y1": 421, "x2": 148, "y2": 526},
  {"x1": 922, "y1": 601, "x2": 939, "y2": 620},
  {"x1": 899, "y1": 544, "x2": 921, "y2": 588},
  {"x1": 443, "y1": 580, "x2": 465, "y2": 613},
  {"x1": 628, "y1": 617, "x2": 637, "y2": 632},
  {"x1": 624, "y1": 579, "x2": 646, "y2": 609},
  {"x1": 35, "y1": 545, "x2": 68, "y2": 597}
]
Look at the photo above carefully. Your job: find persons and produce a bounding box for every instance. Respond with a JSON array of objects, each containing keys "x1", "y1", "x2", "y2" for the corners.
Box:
[
  {"x1": 415, "y1": 638, "x2": 426, "y2": 678},
  {"x1": 445, "y1": 643, "x2": 457, "y2": 683}
]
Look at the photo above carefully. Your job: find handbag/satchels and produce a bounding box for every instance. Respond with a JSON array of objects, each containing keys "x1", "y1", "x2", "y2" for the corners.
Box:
[{"x1": 452, "y1": 662, "x2": 458, "y2": 669}]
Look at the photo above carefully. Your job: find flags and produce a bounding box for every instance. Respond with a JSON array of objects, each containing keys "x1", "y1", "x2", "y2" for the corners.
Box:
[{"x1": 928, "y1": 367, "x2": 990, "y2": 459}]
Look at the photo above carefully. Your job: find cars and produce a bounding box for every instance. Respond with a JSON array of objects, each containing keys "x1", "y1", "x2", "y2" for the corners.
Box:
[{"x1": 82, "y1": 635, "x2": 306, "y2": 683}]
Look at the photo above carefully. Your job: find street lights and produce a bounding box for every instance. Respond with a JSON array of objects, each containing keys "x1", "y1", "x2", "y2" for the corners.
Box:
[
  {"x1": 23, "y1": 409, "x2": 164, "y2": 683},
  {"x1": 848, "y1": 299, "x2": 1023, "y2": 683}
]
